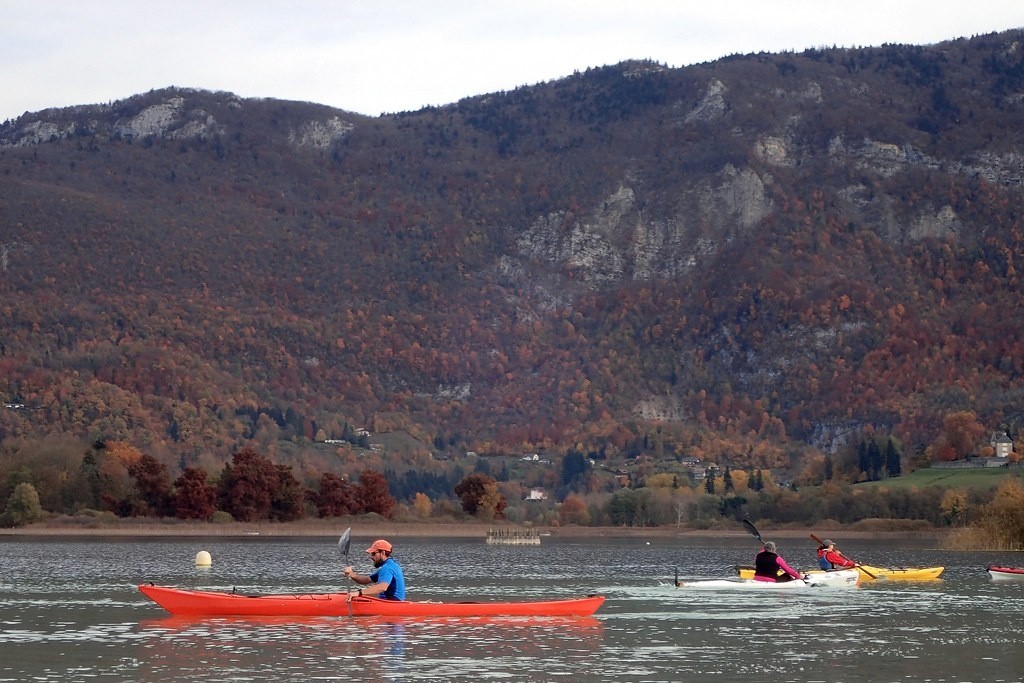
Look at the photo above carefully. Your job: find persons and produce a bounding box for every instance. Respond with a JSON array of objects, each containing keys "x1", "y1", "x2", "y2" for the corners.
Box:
[
  {"x1": 344, "y1": 540, "x2": 406, "y2": 601},
  {"x1": 753, "y1": 541, "x2": 806, "y2": 582},
  {"x1": 816, "y1": 539, "x2": 860, "y2": 571}
]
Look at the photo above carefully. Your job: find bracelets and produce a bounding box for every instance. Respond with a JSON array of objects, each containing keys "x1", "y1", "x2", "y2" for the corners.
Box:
[{"x1": 357, "y1": 589, "x2": 362, "y2": 596}]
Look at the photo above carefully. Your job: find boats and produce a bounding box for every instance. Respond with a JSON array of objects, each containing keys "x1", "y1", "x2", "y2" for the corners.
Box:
[
  {"x1": 138, "y1": 582, "x2": 606, "y2": 617},
  {"x1": 675, "y1": 567, "x2": 859, "y2": 589},
  {"x1": 739, "y1": 567, "x2": 944, "y2": 582},
  {"x1": 987, "y1": 568, "x2": 1024, "y2": 580}
]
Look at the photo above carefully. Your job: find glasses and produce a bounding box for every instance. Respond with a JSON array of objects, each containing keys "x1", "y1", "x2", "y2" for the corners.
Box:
[{"x1": 371, "y1": 551, "x2": 380, "y2": 555}]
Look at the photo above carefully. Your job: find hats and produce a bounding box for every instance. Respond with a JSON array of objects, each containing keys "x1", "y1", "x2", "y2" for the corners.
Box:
[
  {"x1": 822, "y1": 539, "x2": 836, "y2": 546},
  {"x1": 365, "y1": 539, "x2": 393, "y2": 553}
]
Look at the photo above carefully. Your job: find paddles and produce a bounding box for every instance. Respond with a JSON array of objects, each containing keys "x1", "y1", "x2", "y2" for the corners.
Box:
[
  {"x1": 810, "y1": 534, "x2": 880, "y2": 579},
  {"x1": 338, "y1": 526, "x2": 354, "y2": 619},
  {"x1": 742, "y1": 517, "x2": 811, "y2": 585}
]
